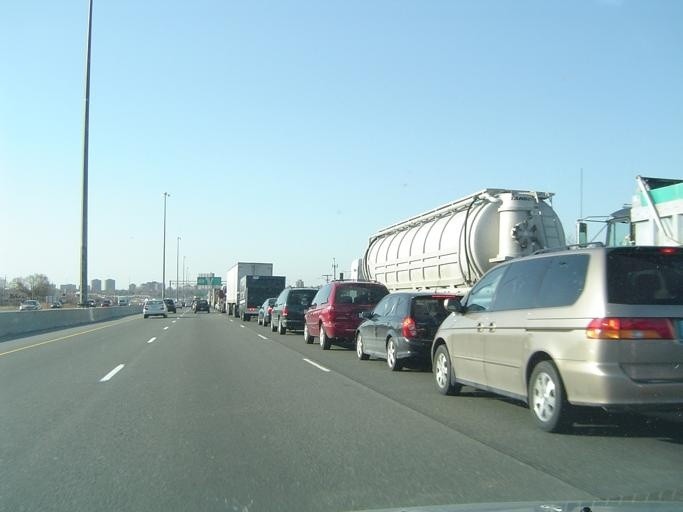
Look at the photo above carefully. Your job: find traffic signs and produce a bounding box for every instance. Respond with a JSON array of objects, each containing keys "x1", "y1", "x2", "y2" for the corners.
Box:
[{"x1": 197, "y1": 276, "x2": 222, "y2": 286}]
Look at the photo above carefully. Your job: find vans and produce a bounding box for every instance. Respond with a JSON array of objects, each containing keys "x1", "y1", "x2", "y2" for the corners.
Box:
[
  {"x1": 118, "y1": 296, "x2": 130, "y2": 306},
  {"x1": 163, "y1": 298, "x2": 177, "y2": 313},
  {"x1": 431, "y1": 242, "x2": 681, "y2": 431},
  {"x1": 270, "y1": 285, "x2": 320, "y2": 334},
  {"x1": 303, "y1": 279, "x2": 389, "y2": 350}
]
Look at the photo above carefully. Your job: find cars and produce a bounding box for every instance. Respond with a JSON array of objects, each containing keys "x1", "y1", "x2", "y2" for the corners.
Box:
[
  {"x1": 50, "y1": 301, "x2": 63, "y2": 308},
  {"x1": 175, "y1": 299, "x2": 183, "y2": 309},
  {"x1": 101, "y1": 299, "x2": 113, "y2": 307},
  {"x1": 143, "y1": 299, "x2": 168, "y2": 319},
  {"x1": 185, "y1": 294, "x2": 210, "y2": 313},
  {"x1": 257, "y1": 297, "x2": 277, "y2": 327},
  {"x1": 20, "y1": 300, "x2": 42, "y2": 312},
  {"x1": 88, "y1": 299, "x2": 97, "y2": 307},
  {"x1": 354, "y1": 291, "x2": 461, "y2": 372}
]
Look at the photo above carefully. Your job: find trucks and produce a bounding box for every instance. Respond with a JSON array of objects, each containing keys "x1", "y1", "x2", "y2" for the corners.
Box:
[
  {"x1": 207, "y1": 261, "x2": 286, "y2": 323},
  {"x1": 350, "y1": 188, "x2": 565, "y2": 300}
]
